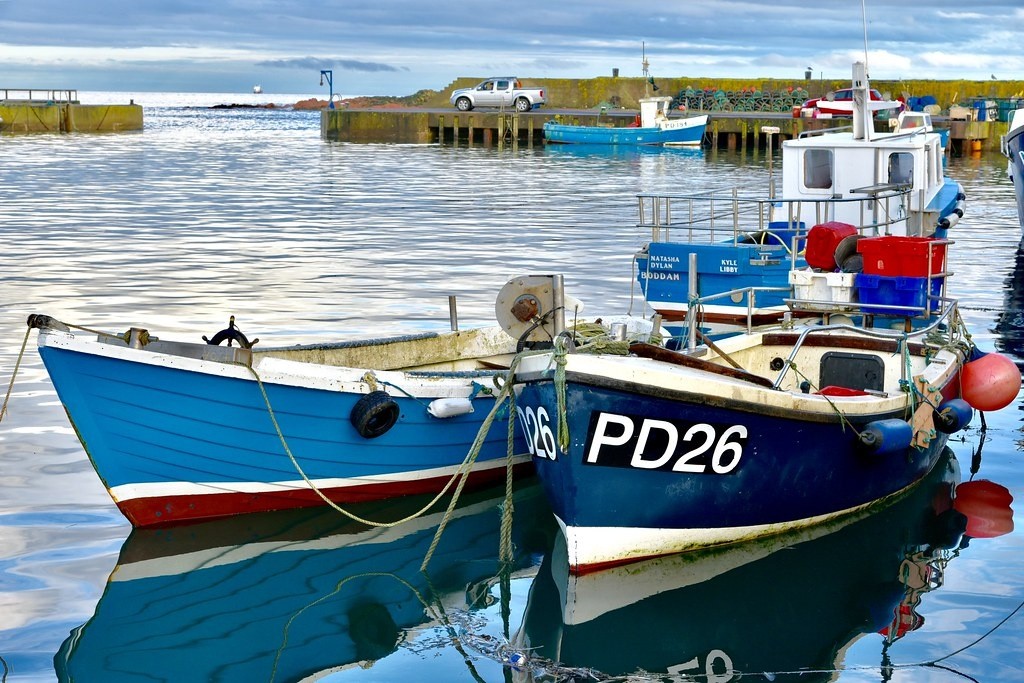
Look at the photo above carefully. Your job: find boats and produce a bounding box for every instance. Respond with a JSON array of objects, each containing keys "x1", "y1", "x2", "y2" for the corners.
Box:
[
  {"x1": 51, "y1": 466, "x2": 559, "y2": 683},
  {"x1": 497, "y1": 221, "x2": 1024, "y2": 577},
  {"x1": 631, "y1": 0, "x2": 966, "y2": 335},
  {"x1": 520, "y1": 450, "x2": 1017, "y2": 682},
  {"x1": 1000, "y1": 107, "x2": 1024, "y2": 227},
  {"x1": 542, "y1": 80, "x2": 709, "y2": 146},
  {"x1": 24, "y1": 311, "x2": 764, "y2": 528}
]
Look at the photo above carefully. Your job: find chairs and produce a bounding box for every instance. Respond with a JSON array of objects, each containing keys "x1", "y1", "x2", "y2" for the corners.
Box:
[{"x1": 819, "y1": 351, "x2": 884, "y2": 392}]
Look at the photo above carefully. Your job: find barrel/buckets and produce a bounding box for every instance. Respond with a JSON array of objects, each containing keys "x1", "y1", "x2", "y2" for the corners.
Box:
[
  {"x1": 971, "y1": 140, "x2": 982, "y2": 152},
  {"x1": 975, "y1": 102, "x2": 986, "y2": 121},
  {"x1": 770, "y1": 221, "x2": 806, "y2": 253},
  {"x1": 908, "y1": 96, "x2": 941, "y2": 113},
  {"x1": 835, "y1": 234, "x2": 865, "y2": 271}
]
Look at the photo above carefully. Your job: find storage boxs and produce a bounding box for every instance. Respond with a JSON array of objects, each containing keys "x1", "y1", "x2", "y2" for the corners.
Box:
[{"x1": 788, "y1": 221, "x2": 948, "y2": 316}]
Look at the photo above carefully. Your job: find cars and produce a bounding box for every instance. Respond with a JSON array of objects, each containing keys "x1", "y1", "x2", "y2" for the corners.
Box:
[{"x1": 802, "y1": 87, "x2": 883, "y2": 118}]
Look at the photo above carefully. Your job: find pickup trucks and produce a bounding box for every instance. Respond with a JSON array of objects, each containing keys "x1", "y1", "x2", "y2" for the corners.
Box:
[{"x1": 450, "y1": 76, "x2": 548, "y2": 112}]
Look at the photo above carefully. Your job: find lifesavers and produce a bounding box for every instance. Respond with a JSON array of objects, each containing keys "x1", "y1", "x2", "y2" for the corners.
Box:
[
  {"x1": 834, "y1": 235, "x2": 867, "y2": 269},
  {"x1": 350, "y1": 603, "x2": 398, "y2": 657},
  {"x1": 350, "y1": 391, "x2": 400, "y2": 439}
]
